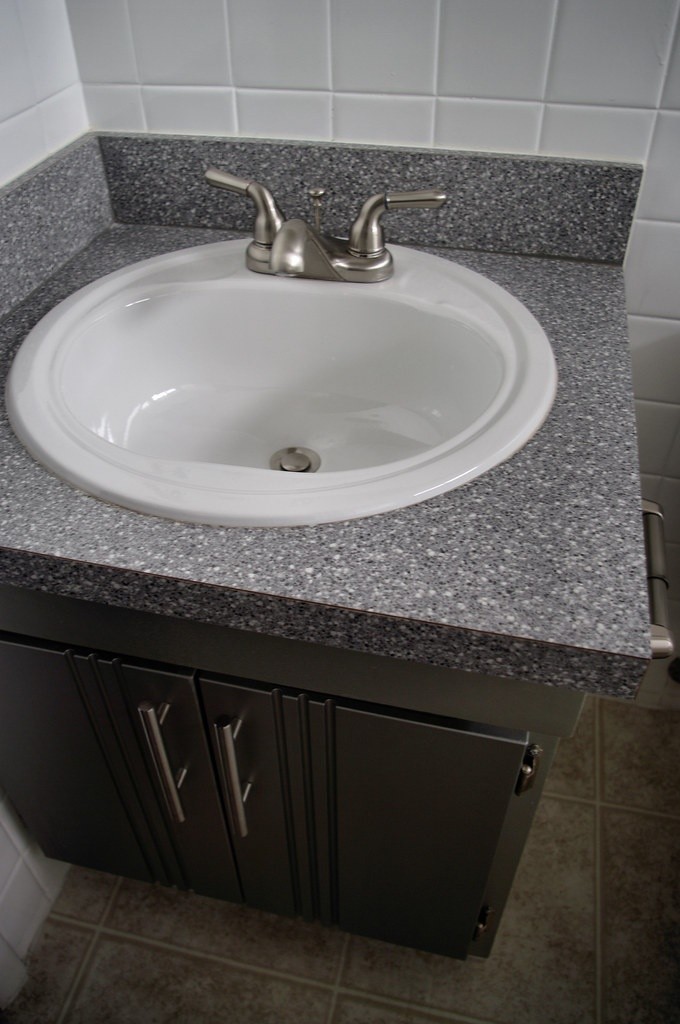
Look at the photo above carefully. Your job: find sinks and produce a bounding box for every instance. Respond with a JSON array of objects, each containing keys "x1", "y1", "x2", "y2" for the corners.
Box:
[{"x1": 5, "y1": 238, "x2": 558, "y2": 527}]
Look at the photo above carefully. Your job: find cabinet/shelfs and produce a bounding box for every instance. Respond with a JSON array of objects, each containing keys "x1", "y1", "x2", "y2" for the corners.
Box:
[{"x1": 1, "y1": 584, "x2": 592, "y2": 959}]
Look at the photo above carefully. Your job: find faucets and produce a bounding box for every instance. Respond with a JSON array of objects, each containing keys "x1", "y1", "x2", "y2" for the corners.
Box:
[{"x1": 268, "y1": 218, "x2": 348, "y2": 281}]
